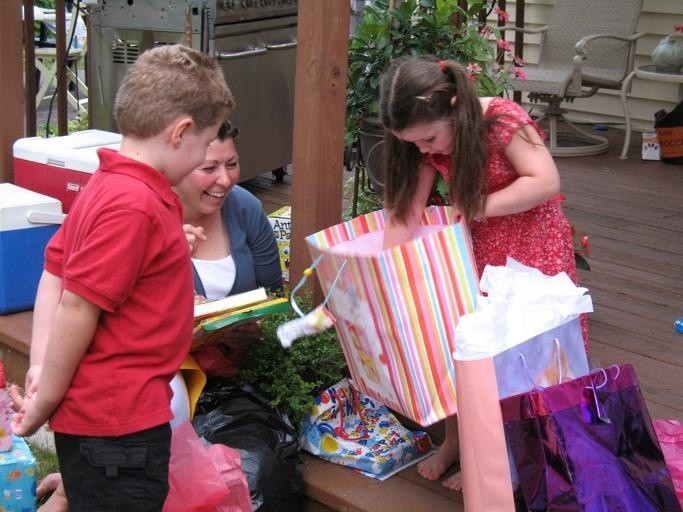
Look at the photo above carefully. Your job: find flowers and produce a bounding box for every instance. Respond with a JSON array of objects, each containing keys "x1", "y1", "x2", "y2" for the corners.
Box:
[{"x1": 465, "y1": 9, "x2": 526, "y2": 98}]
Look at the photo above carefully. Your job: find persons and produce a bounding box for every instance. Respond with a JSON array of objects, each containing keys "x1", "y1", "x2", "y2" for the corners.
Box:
[
  {"x1": 381, "y1": 55, "x2": 589, "y2": 494},
  {"x1": 36, "y1": 119, "x2": 286, "y2": 511},
  {"x1": 8, "y1": 45, "x2": 236, "y2": 512}
]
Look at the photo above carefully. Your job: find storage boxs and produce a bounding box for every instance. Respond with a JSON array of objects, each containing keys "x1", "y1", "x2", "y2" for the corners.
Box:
[
  {"x1": 12, "y1": 128, "x2": 124, "y2": 214},
  {"x1": 0, "y1": 182, "x2": 67, "y2": 316}
]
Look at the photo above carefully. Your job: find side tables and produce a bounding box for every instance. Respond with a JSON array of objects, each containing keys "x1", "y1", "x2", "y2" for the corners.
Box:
[{"x1": 618, "y1": 62, "x2": 683, "y2": 160}]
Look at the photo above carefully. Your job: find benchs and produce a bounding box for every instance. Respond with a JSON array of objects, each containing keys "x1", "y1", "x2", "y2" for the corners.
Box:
[{"x1": 0, "y1": 302, "x2": 463, "y2": 511}]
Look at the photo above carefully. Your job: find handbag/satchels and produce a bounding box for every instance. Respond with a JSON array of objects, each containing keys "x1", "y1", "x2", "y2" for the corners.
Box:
[
  {"x1": 293, "y1": 205, "x2": 481, "y2": 427},
  {"x1": 499, "y1": 362, "x2": 683, "y2": 512},
  {"x1": 650, "y1": 416, "x2": 683, "y2": 510},
  {"x1": 455, "y1": 314, "x2": 591, "y2": 512},
  {"x1": 299, "y1": 377, "x2": 435, "y2": 475}
]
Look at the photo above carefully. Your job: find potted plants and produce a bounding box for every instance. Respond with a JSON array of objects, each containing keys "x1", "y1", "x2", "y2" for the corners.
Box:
[{"x1": 343, "y1": 1, "x2": 486, "y2": 196}]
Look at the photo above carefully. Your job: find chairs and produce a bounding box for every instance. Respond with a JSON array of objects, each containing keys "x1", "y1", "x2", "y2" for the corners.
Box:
[
  {"x1": 492, "y1": 0, "x2": 643, "y2": 158},
  {"x1": 29, "y1": 1, "x2": 88, "y2": 119}
]
